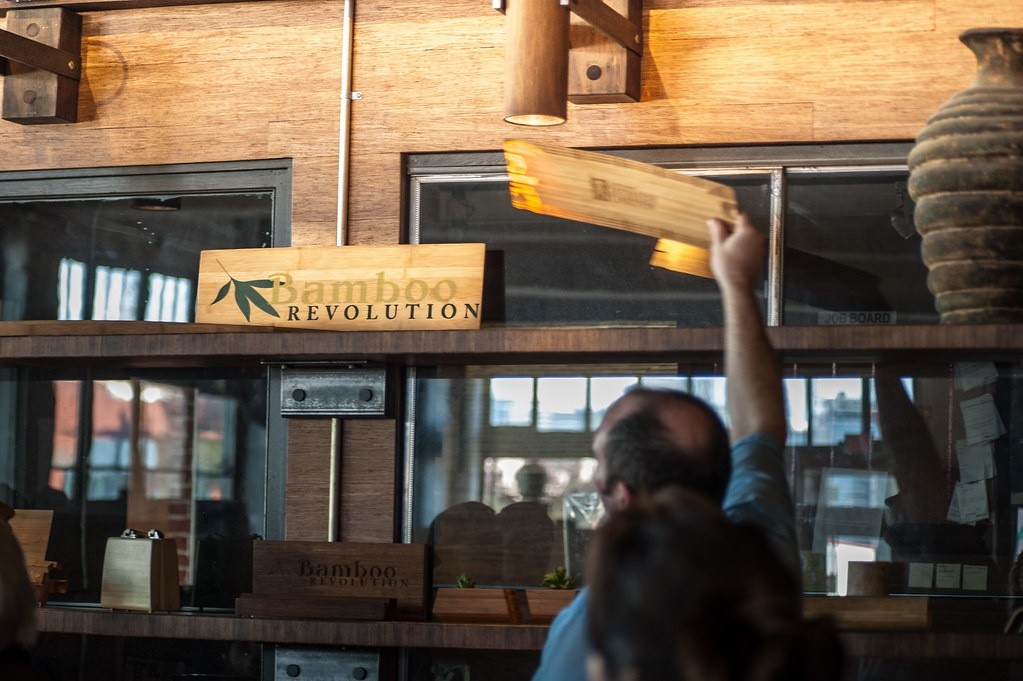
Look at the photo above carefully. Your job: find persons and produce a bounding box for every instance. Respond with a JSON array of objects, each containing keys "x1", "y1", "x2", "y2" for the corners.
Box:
[
  {"x1": 0, "y1": 499, "x2": 41, "y2": 651},
  {"x1": 532, "y1": 206, "x2": 801, "y2": 681},
  {"x1": 584, "y1": 488, "x2": 803, "y2": 681}
]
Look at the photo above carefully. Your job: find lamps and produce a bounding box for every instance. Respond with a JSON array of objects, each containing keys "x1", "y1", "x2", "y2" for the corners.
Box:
[
  {"x1": 0, "y1": 7, "x2": 82, "y2": 124},
  {"x1": 890, "y1": 181, "x2": 916, "y2": 239},
  {"x1": 492, "y1": 0, "x2": 642, "y2": 126}
]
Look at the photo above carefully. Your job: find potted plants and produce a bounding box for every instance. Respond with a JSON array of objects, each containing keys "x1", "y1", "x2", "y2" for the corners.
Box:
[
  {"x1": 432, "y1": 572, "x2": 523, "y2": 625},
  {"x1": 525, "y1": 567, "x2": 579, "y2": 623}
]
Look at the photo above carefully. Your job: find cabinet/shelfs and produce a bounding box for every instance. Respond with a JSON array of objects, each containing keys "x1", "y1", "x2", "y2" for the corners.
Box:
[{"x1": 0, "y1": 326, "x2": 1022, "y2": 650}]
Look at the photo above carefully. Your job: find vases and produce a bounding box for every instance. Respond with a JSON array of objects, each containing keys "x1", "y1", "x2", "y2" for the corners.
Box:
[{"x1": 908, "y1": 26, "x2": 1023, "y2": 326}]
